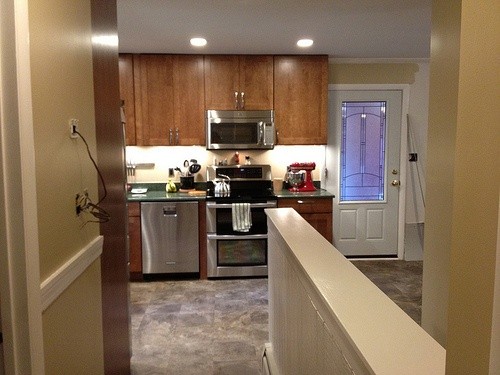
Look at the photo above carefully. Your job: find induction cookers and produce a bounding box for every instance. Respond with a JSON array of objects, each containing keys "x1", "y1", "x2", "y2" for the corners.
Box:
[{"x1": 206, "y1": 164, "x2": 276, "y2": 199}]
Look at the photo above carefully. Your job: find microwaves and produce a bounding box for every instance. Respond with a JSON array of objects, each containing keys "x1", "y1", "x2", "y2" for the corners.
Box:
[{"x1": 205, "y1": 109, "x2": 276, "y2": 151}]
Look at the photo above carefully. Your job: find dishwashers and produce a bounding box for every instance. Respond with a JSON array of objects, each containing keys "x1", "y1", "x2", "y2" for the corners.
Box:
[{"x1": 140, "y1": 201, "x2": 200, "y2": 277}]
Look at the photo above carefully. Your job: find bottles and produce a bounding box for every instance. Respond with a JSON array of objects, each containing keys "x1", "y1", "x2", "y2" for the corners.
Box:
[
  {"x1": 214, "y1": 156, "x2": 228, "y2": 165},
  {"x1": 165, "y1": 168, "x2": 176, "y2": 193},
  {"x1": 244, "y1": 155, "x2": 252, "y2": 164},
  {"x1": 235, "y1": 152, "x2": 240, "y2": 164}
]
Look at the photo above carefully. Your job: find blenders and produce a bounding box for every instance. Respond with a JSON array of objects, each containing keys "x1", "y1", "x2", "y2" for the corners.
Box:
[{"x1": 289, "y1": 162, "x2": 318, "y2": 192}]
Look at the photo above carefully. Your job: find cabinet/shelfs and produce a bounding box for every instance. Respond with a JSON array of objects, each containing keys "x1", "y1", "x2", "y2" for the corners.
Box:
[
  {"x1": 202, "y1": 54, "x2": 274, "y2": 111},
  {"x1": 271, "y1": 53, "x2": 327, "y2": 146},
  {"x1": 127, "y1": 200, "x2": 142, "y2": 281},
  {"x1": 132, "y1": 54, "x2": 206, "y2": 148},
  {"x1": 276, "y1": 197, "x2": 333, "y2": 247},
  {"x1": 117, "y1": 52, "x2": 135, "y2": 147}
]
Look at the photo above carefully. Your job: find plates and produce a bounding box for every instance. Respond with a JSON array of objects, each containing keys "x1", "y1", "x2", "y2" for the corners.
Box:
[{"x1": 131, "y1": 188, "x2": 147, "y2": 194}]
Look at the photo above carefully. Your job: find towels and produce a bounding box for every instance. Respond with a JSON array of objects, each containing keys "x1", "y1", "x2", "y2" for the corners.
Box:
[{"x1": 231, "y1": 202, "x2": 253, "y2": 233}]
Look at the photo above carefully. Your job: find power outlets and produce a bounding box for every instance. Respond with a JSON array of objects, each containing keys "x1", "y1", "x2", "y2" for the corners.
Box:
[
  {"x1": 408, "y1": 152, "x2": 418, "y2": 163},
  {"x1": 68, "y1": 117, "x2": 79, "y2": 139}
]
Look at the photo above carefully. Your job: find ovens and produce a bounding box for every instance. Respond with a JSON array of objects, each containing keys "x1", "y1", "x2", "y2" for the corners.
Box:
[{"x1": 206, "y1": 196, "x2": 277, "y2": 278}]
